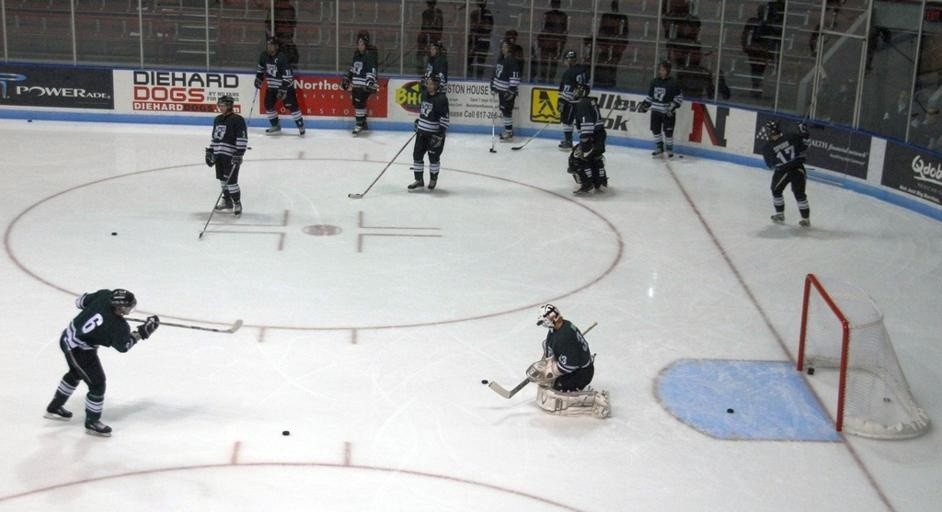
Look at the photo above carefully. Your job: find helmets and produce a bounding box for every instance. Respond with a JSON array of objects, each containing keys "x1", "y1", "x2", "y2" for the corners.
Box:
[
  {"x1": 424, "y1": 75, "x2": 441, "y2": 93},
  {"x1": 217, "y1": 96, "x2": 234, "y2": 113},
  {"x1": 429, "y1": 43, "x2": 442, "y2": 56},
  {"x1": 264, "y1": 36, "x2": 280, "y2": 54},
  {"x1": 535, "y1": 303, "x2": 563, "y2": 329},
  {"x1": 765, "y1": 120, "x2": 780, "y2": 138},
  {"x1": 108, "y1": 288, "x2": 138, "y2": 315},
  {"x1": 572, "y1": 83, "x2": 591, "y2": 100},
  {"x1": 356, "y1": 33, "x2": 369, "y2": 45},
  {"x1": 502, "y1": 41, "x2": 513, "y2": 54},
  {"x1": 563, "y1": 49, "x2": 577, "y2": 63}
]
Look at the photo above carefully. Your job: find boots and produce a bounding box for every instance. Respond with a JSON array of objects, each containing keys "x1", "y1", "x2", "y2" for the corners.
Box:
[
  {"x1": 499, "y1": 131, "x2": 514, "y2": 143},
  {"x1": 42, "y1": 398, "x2": 73, "y2": 422},
  {"x1": 558, "y1": 140, "x2": 574, "y2": 151},
  {"x1": 265, "y1": 123, "x2": 283, "y2": 136},
  {"x1": 651, "y1": 146, "x2": 675, "y2": 161},
  {"x1": 571, "y1": 177, "x2": 606, "y2": 196},
  {"x1": 211, "y1": 198, "x2": 243, "y2": 219},
  {"x1": 297, "y1": 122, "x2": 306, "y2": 138},
  {"x1": 84, "y1": 413, "x2": 113, "y2": 438},
  {"x1": 407, "y1": 178, "x2": 425, "y2": 191},
  {"x1": 351, "y1": 122, "x2": 369, "y2": 137},
  {"x1": 427, "y1": 175, "x2": 438, "y2": 191},
  {"x1": 770, "y1": 212, "x2": 785, "y2": 225},
  {"x1": 798, "y1": 217, "x2": 813, "y2": 231}
]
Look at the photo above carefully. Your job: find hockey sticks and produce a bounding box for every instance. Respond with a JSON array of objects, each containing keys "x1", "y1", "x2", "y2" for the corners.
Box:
[
  {"x1": 488, "y1": 323, "x2": 596, "y2": 399},
  {"x1": 511, "y1": 110, "x2": 558, "y2": 151},
  {"x1": 802, "y1": 66, "x2": 826, "y2": 126},
  {"x1": 347, "y1": 134, "x2": 415, "y2": 199},
  {"x1": 198, "y1": 164, "x2": 235, "y2": 239},
  {"x1": 126, "y1": 317, "x2": 244, "y2": 333},
  {"x1": 491, "y1": 96, "x2": 498, "y2": 154}
]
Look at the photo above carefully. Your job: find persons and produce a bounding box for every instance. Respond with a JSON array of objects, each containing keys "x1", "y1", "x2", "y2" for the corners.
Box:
[
  {"x1": 259, "y1": 2, "x2": 786, "y2": 104},
  {"x1": 528, "y1": 304, "x2": 597, "y2": 392},
  {"x1": 555, "y1": 48, "x2": 588, "y2": 148},
  {"x1": 47, "y1": 287, "x2": 160, "y2": 435},
  {"x1": 637, "y1": 59, "x2": 682, "y2": 159},
  {"x1": 204, "y1": 94, "x2": 248, "y2": 218},
  {"x1": 758, "y1": 118, "x2": 812, "y2": 229},
  {"x1": 404, "y1": 73, "x2": 451, "y2": 190},
  {"x1": 341, "y1": 36, "x2": 380, "y2": 136},
  {"x1": 253, "y1": 35, "x2": 306, "y2": 137},
  {"x1": 564, "y1": 84, "x2": 611, "y2": 193},
  {"x1": 805, "y1": 61, "x2": 941, "y2": 157},
  {"x1": 489, "y1": 37, "x2": 519, "y2": 140},
  {"x1": 424, "y1": 41, "x2": 449, "y2": 99}
]
